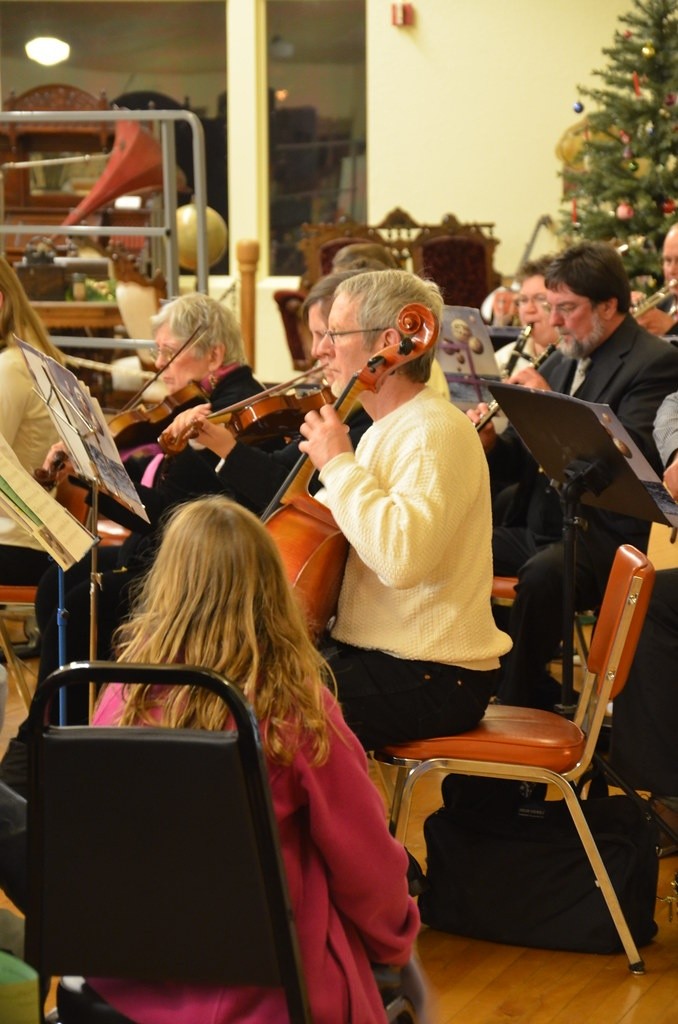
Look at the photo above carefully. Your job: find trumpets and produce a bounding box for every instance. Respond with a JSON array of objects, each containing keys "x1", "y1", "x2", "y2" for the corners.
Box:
[{"x1": 631, "y1": 277, "x2": 678, "y2": 319}]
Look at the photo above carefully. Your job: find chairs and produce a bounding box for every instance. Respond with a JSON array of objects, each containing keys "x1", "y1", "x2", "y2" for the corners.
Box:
[
  {"x1": 0, "y1": 585, "x2": 38, "y2": 714},
  {"x1": 25, "y1": 660, "x2": 313, "y2": 1024},
  {"x1": 274, "y1": 207, "x2": 504, "y2": 371},
  {"x1": 369, "y1": 542, "x2": 647, "y2": 976},
  {"x1": 491, "y1": 576, "x2": 589, "y2": 663}
]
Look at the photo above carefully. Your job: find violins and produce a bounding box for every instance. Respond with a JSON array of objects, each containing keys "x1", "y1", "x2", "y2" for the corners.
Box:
[
  {"x1": 157, "y1": 386, "x2": 338, "y2": 456},
  {"x1": 31, "y1": 379, "x2": 210, "y2": 487}
]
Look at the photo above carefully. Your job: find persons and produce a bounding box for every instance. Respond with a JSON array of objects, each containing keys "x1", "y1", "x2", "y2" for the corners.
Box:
[
  {"x1": 1, "y1": 221, "x2": 678, "y2": 856},
  {"x1": 86, "y1": 498, "x2": 424, "y2": 1023},
  {"x1": 298, "y1": 274, "x2": 512, "y2": 752},
  {"x1": 0, "y1": 252, "x2": 72, "y2": 585}
]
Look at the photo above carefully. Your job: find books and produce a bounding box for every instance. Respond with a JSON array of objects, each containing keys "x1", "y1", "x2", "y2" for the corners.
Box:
[
  {"x1": 478, "y1": 378, "x2": 677, "y2": 528},
  {"x1": 11, "y1": 332, "x2": 150, "y2": 523},
  {"x1": 1, "y1": 434, "x2": 98, "y2": 570},
  {"x1": 433, "y1": 305, "x2": 505, "y2": 401}
]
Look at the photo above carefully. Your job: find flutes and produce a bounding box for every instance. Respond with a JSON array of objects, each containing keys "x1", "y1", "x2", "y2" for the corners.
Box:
[{"x1": 472, "y1": 335, "x2": 564, "y2": 432}]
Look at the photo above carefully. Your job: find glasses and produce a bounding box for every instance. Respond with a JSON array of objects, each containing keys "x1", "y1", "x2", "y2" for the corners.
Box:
[
  {"x1": 657, "y1": 256, "x2": 678, "y2": 268},
  {"x1": 148, "y1": 345, "x2": 179, "y2": 364},
  {"x1": 323, "y1": 326, "x2": 392, "y2": 345},
  {"x1": 541, "y1": 299, "x2": 590, "y2": 314}
]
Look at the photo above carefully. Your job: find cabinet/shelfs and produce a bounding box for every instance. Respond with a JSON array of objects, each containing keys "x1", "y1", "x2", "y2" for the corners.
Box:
[{"x1": 0, "y1": 84, "x2": 115, "y2": 253}]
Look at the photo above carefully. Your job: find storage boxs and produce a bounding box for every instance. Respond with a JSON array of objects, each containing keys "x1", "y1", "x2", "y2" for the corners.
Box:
[
  {"x1": 13, "y1": 261, "x2": 65, "y2": 302},
  {"x1": 52, "y1": 257, "x2": 110, "y2": 282}
]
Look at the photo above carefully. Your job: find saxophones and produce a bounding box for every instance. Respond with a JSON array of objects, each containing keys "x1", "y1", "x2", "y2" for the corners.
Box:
[{"x1": 502, "y1": 321, "x2": 536, "y2": 378}]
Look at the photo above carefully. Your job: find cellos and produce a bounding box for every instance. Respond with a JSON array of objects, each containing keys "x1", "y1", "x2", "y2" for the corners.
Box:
[{"x1": 259, "y1": 298, "x2": 439, "y2": 655}]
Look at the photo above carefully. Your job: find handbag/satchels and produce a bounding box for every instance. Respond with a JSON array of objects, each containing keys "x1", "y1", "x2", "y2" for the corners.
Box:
[{"x1": 416, "y1": 793, "x2": 661, "y2": 951}]
